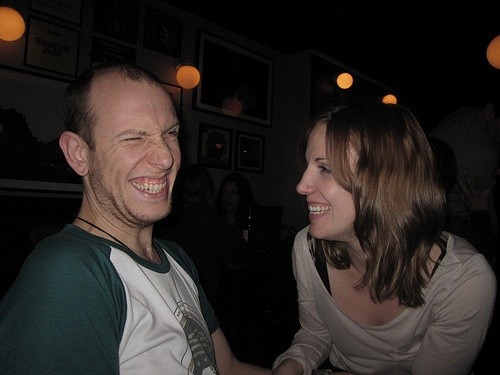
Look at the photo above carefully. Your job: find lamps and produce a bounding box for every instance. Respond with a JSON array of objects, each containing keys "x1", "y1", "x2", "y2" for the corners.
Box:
[
  {"x1": 176, "y1": 57, "x2": 200, "y2": 89},
  {"x1": 0, "y1": 0, "x2": 27, "y2": 42}
]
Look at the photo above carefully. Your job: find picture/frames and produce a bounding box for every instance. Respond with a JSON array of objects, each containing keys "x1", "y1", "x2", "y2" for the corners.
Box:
[
  {"x1": 192, "y1": 25, "x2": 276, "y2": 128},
  {"x1": 89, "y1": 35, "x2": 137, "y2": 69},
  {"x1": 235, "y1": 130, "x2": 265, "y2": 174},
  {"x1": 28, "y1": 0, "x2": 84, "y2": 27},
  {"x1": 23, "y1": 15, "x2": 81, "y2": 77},
  {"x1": 197, "y1": 121, "x2": 234, "y2": 170},
  {"x1": 92, "y1": 0, "x2": 141, "y2": 42},
  {"x1": 143, "y1": 5, "x2": 185, "y2": 59}
]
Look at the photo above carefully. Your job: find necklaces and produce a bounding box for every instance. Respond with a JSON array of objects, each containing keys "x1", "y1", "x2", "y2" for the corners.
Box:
[{"x1": 72, "y1": 216, "x2": 165, "y2": 263}]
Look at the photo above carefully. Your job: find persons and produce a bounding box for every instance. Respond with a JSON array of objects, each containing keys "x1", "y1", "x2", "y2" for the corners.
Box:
[
  {"x1": 216, "y1": 172, "x2": 277, "y2": 256},
  {"x1": 271, "y1": 100, "x2": 497, "y2": 375},
  {"x1": 1, "y1": 60, "x2": 273, "y2": 374},
  {"x1": 428, "y1": 96, "x2": 500, "y2": 272},
  {"x1": 152, "y1": 164, "x2": 257, "y2": 307}
]
